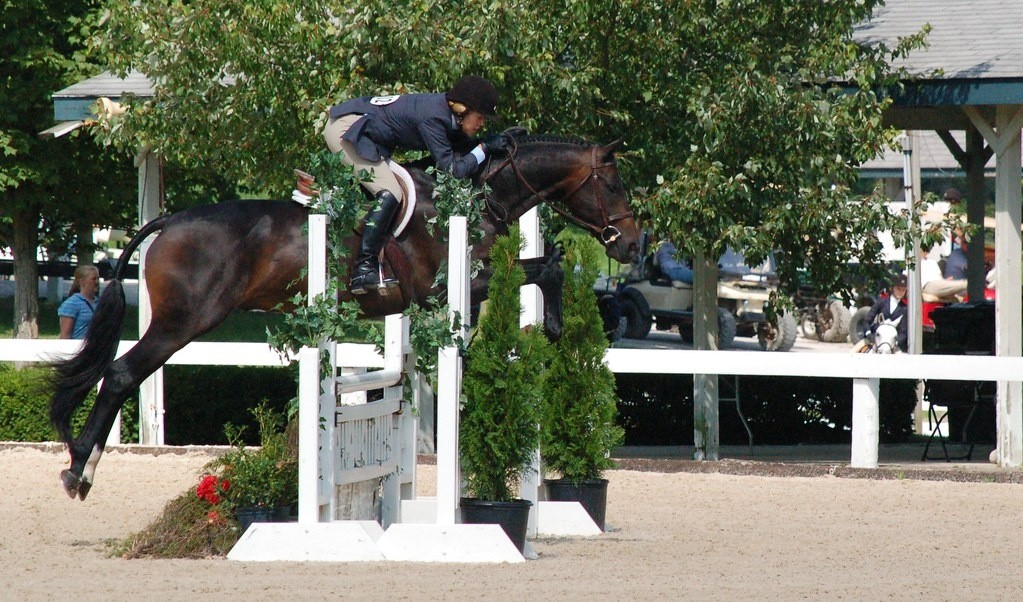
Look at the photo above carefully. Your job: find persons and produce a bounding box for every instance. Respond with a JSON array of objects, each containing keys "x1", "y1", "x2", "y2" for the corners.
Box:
[
  {"x1": 852, "y1": 189, "x2": 997, "y2": 353},
  {"x1": 58, "y1": 265, "x2": 101, "y2": 339},
  {"x1": 324, "y1": 76, "x2": 508, "y2": 295}
]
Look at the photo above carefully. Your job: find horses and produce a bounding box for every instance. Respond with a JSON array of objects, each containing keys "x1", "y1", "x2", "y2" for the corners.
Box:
[{"x1": 35, "y1": 133, "x2": 644, "y2": 502}]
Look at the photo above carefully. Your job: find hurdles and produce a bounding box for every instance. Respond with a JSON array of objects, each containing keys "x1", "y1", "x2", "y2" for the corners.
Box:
[{"x1": 224, "y1": 202, "x2": 603, "y2": 566}]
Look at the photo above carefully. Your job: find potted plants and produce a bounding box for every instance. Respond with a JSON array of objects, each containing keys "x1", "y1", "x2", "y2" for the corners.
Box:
[
  {"x1": 543, "y1": 232, "x2": 625, "y2": 532},
  {"x1": 461, "y1": 224, "x2": 558, "y2": 557}
]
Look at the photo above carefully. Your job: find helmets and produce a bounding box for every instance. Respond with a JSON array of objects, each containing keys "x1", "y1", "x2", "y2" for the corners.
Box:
[
  {"x1": 890, "y1": 274, "x2": 906, "y2": 287},
  {"x1": 445, "y1": 75, "x2": 497, "y2": 116}
]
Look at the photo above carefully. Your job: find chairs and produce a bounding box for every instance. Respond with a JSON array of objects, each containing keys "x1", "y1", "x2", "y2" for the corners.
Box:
[{"x1": 921, "y1": 301, "x2": 996, "y2": 463}]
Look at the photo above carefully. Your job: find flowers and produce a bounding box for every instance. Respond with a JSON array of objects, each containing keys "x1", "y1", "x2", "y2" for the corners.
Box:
[{"x1": 195, "y1": 400, "x2": 298, "y2": 520}]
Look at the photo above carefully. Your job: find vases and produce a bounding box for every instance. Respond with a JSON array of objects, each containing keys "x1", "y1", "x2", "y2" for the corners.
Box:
[{"x1": 234, "y1": 503, "x2": 297, "y2": 536}]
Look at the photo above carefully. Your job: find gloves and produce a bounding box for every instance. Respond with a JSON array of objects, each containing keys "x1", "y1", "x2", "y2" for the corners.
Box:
[
  {"x1": 866, "y1": 333, "x2": 873, "y2": 344},
  {"x1": 481, "y1": 134, "x2": 509, "y2": 157},
  {"x1": 506, "y1": 128, "x2": 527, "y2": 136}
]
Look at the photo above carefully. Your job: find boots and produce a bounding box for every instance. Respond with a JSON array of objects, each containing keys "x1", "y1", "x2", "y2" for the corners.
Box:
[{"x1": 349, "y1": 189, "x2": 400, "y2": 296}]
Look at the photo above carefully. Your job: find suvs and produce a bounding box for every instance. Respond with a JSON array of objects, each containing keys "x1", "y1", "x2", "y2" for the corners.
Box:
[
  {"x1": 594, "y1": 234, "x2": 799, "y2": 353},
  {"x1": 799, "y1": 198, "x2": 955, "y2": 346}
]
